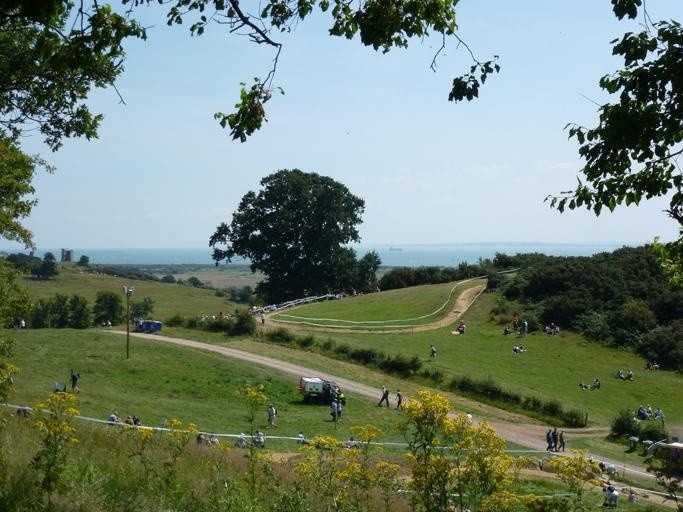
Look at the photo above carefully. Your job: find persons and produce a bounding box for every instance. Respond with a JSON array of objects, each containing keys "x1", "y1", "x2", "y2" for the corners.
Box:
[
  {"x1": 603, "y1": 486, "x2": 620, "y2": 509},
  {"x1": 557, "y1": 429, "x2": 565, "y2": 452},
  {"x1": 456, "y1": 321, "x2": 465, "y2": 334},
  {"x1": 551, "y1": 428, "x2": 558, "y2": 452},
  {"x1": 504, "y1": 315, "x2": 560, "y2": 353},
  {"x1": 330, "y1": 398, "x2": 338, "y2": 422},
  {"x1": 124, "y1": 415, "x2": 133, "y2": 425},
  {"x1": 200, "y1": 304, "x2": 277, "y2": 327},
  {"x1": 20, "y1": 319, "x2": 25, "y2": 328},
  {"x1": 335, "y1": 286, "x2": 381, "y2": 302},
  {"x1": 394, "y1": 389, "x2": 403, "y2": 410},
  {"x1": 133, "y1": 415, "x2": 141, "y2": 426},
  {"x1": 466, "y1": 410, "x2": 472, "y2": 420},
  {"x1": 106, "y1": 319, "x2": 111, "y2": 328},
  {"x1": 196, "y1": 428, "x2": 266, "y2": 449},
  {"x1": 100, "y1": 321, "x2": 106, "y2": 329},
  {"x1": 296, "y1": 431, "x2": 304, "y2": 444},
  {"x1": 107, "y1": 411, "x2": 120, "y2": 426},
  {"x1": 70, "y1": 368, "x2": 80, "y2": 394},
  {"x1": 137, "y1": 318, "x2": 145, "y2": 332},
  {"x1": 628, "y1": 491, "x2": 639, "y2": 503},
  {"x1": 267, "y1": 404, "x2": 277, "y2": 430},
  {"x1": 579, "y1": 360, "x2": 666, "y2": 421},
  {"x1": 545, "y1": 429, "x2": 552, "y2": 450},
  {"x1": 377, "y1": 385, "x2": 389, "y2": 407},
  {"x1": 343, "y1": 437, "x2": 354, "y2": 448},
  {"x1": 337, "y1": 400, "x2": 342, "y2": 422},
  {"x1": 428, "y1": 344, "x2": 437, "y2": 361}
]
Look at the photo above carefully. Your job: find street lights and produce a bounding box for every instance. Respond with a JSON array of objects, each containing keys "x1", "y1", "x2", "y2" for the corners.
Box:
[{"x1": 122, "y1": 285, "x2": 135, "y2": 359}]
